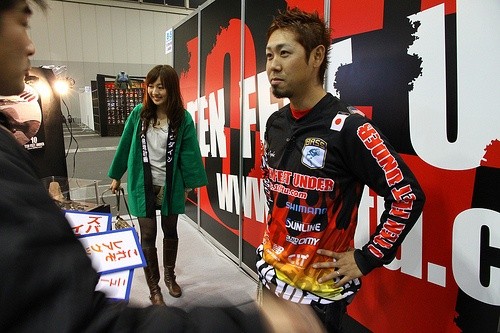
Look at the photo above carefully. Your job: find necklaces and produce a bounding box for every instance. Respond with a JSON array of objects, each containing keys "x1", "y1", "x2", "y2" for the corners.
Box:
[{"x1": 157, "y1": 120, "x2": 160, "y2": 126}]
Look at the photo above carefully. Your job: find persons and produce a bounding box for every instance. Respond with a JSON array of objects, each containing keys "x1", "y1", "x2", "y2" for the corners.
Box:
[
  {"x1": 106, "y1": 65, "x2": 209, "y2": 307},
  {"x1": 255, "y1": 8, "x2": 425, "y2": 333},
  {"x1": 0, "y1": 1, "x2": 325, "y2": 333},
  {"x1": 114, "y1": 72, "x2": 132, "y2": 90}
]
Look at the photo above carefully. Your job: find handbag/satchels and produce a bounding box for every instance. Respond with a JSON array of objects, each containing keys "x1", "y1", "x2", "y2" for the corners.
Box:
[
  {"x1": 115, "y1": 187, "x2": 139, "y2": 239},
  {"x1": 313, "y1": 302, "x2": 340, "y2": 333}
]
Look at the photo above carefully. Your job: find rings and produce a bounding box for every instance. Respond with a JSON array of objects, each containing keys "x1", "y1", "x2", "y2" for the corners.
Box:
[{"x1": 337, "y1": 271, "x2": 340, "y2": 275}]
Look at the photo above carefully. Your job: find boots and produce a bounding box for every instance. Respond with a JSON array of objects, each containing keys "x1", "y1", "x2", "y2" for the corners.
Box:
[
  {"x1": 162, "y1": 238, "x2": 182, "y2": 297},
  {"x1": 141, "y1": 248, "x2": 163, "y2": 305}
]
